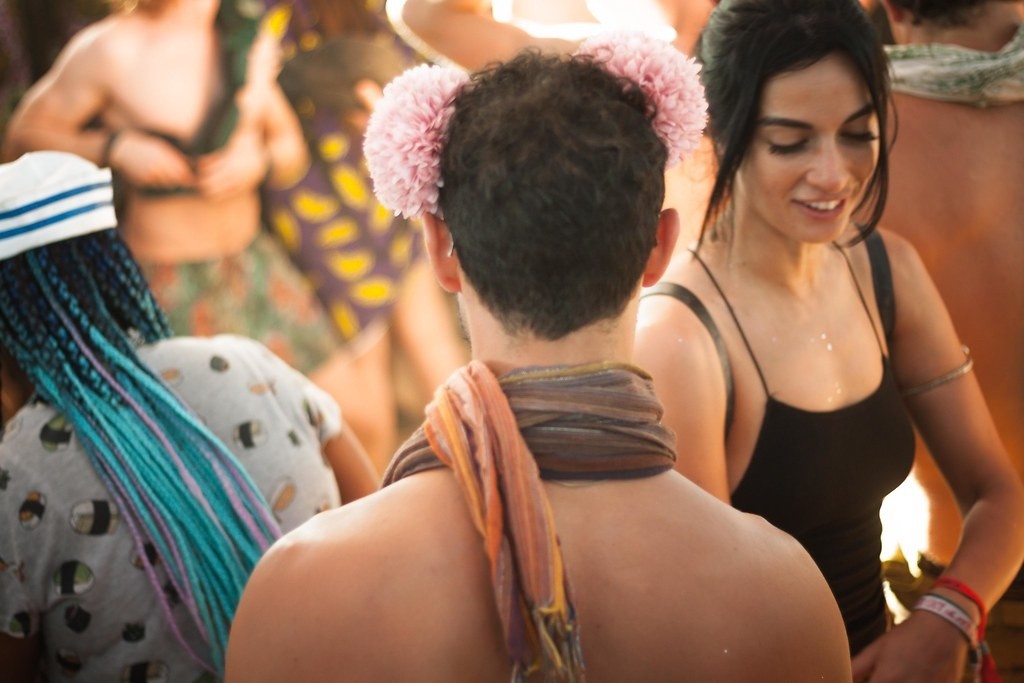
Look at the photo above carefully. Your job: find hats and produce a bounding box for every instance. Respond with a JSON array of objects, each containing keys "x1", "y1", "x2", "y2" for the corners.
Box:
[{"x1": 0, "y1": 147, "x2": 120, "y2": 262}]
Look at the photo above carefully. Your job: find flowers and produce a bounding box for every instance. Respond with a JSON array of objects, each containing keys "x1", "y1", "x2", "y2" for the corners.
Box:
[
  {"x1": 363, "y1": 61, "x2": 473, "y2": 223},
  {"x1": 571, "y1": 29, "x2": 708, "y2": 167}
]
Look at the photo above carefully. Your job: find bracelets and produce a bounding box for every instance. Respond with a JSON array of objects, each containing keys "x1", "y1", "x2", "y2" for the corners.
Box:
[
  {"x1": 913, "y1": 591, "x2": 982, "y2": 683},
  {"x1": 928, "y1": 573, "x2": 1001, "y2": 683},
  {"x1": 100, "y1": 130, "x2": 120, "y2": 167}
]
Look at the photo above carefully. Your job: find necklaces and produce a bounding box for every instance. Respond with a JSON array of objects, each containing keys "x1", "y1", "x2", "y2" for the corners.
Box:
[{"x1": 552, "y1": 479, "x2": 605, "y2": 489}]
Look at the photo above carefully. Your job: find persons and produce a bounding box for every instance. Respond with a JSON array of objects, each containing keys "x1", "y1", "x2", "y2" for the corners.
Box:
[{"x1": 0, "y1": 0, "x2": 1024, "y2": 683}]
